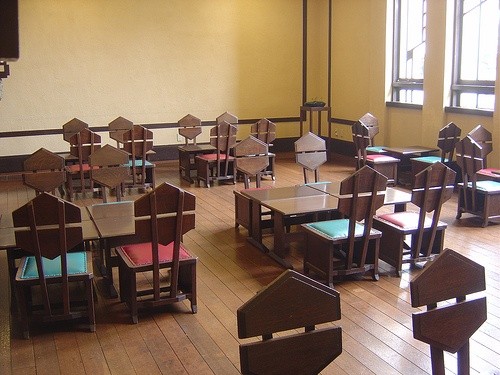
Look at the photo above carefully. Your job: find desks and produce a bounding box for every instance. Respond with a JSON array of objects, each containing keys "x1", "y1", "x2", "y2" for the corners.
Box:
[
  {"x1": 0, "y1": 206, "x2": 101, "y2": 310},
  {"x1": 383, "y1": 145, "x2": 439, "y2": 186},
  {"x1": 52, "y1": 149, "x2": 156, "y2": 195},
  {"x1": 241, "y1": 185, "x2": 338, "y2": 267},
  {"x1": 299, "y1": 106, "x2": 332, "y2": 144},
  {"x1": 87, "y1": 204, "x2": 192, "y2": 300},
  {"x1": 177, "y1": 144, "x2": 273, "y2": 183},
  {"x1": 306, "y1": 181, "x2": 415, "y2": 250}
]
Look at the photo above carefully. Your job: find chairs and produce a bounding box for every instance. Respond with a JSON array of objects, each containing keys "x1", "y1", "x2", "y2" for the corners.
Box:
[{"x1": 14, "y1": 113, "x2": 500, "y2": 375}]
